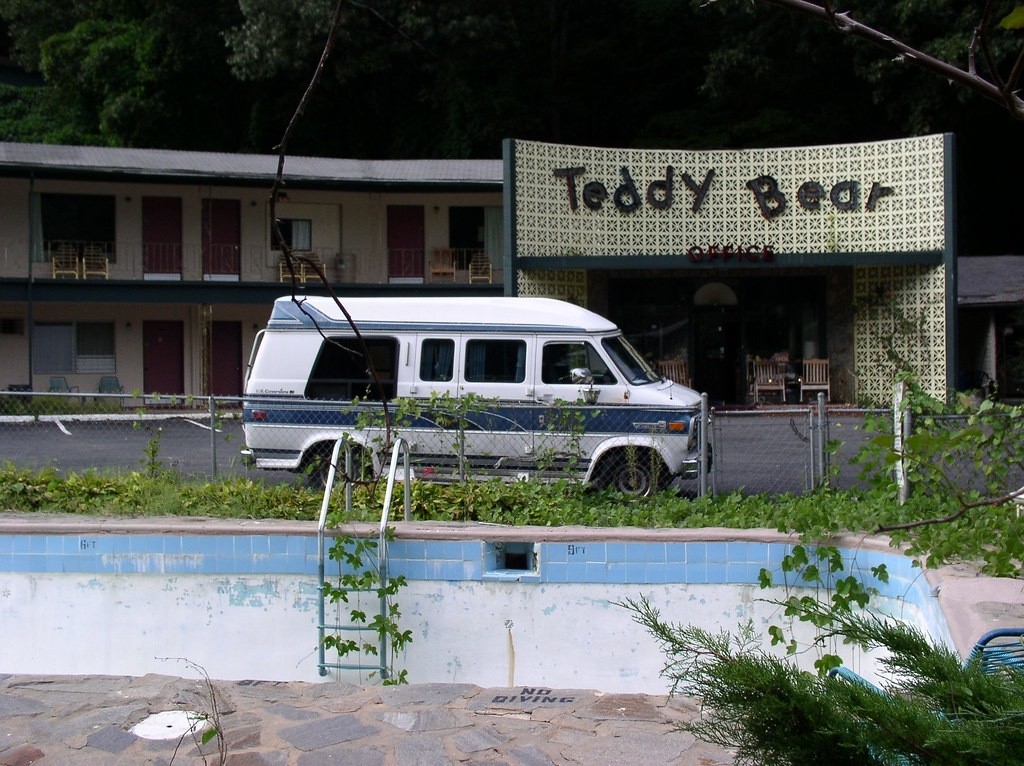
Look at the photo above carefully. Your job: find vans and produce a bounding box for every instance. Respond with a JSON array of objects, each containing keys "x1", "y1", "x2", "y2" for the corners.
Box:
[{"x1": 240, "y1": 294, "x2": 714, "y2": 506}]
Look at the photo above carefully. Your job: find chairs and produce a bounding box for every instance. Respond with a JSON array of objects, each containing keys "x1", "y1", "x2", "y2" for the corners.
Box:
[
  {"x1": 800, "y1": 359, "x2": 831, "y2": 403},
  {"x1": 52, "y1": 243, "x2": 79, "y2": 280},
  {"x1": 745, "y1": 355, "x2": 761, "y2": 396},
  {"x1": 774, "y1": 351, "x2": 789, "y2": 363},
  {"x1": 826, "y1": 626, "x2": 1024, "y2": 766},
  {"x1": 752, "y1": 360, "x2": 786, "y2": 404},
  {"x1": 48, "y1": 376, "x2": 80, "y2": 402},
  {"x1": 83, "y1": 241, "x2": 109, "y2": 279},
  {"x1": 428, "y1": 247, "x2": 456, "y2": 283},
  {"x1": 657, "y1": 359, "x2": 691, "y2": 387},
  {"x1": 94, "y1": 376, "x2": 125, "y2": 411},
  {"x1": 304, "y1": 253, "x2": 326, "y2": 283},
  {"x1": 469, "y1": 253, "x2": 492, "y2": 284},
  {"x1": 278, "y1": 253, "x2": 304, "y2": 283}
]
